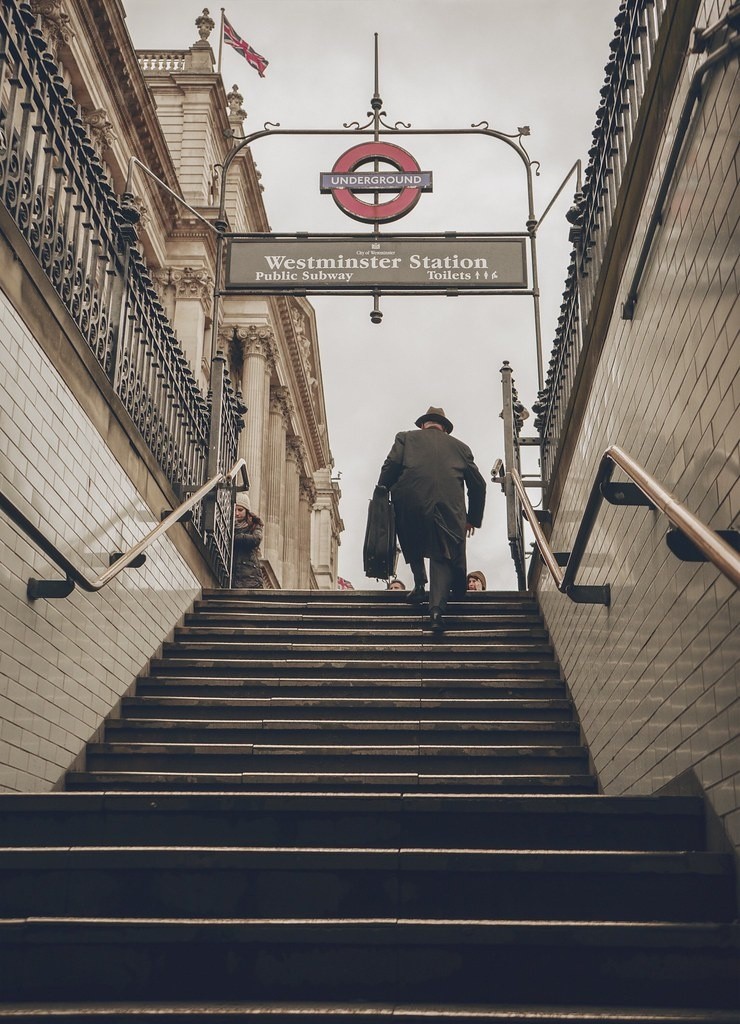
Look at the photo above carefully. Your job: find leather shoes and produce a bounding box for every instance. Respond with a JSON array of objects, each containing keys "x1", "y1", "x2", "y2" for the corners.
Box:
[
  {"x1": 406, "y1": 586, "x2": 426, "y2": 603},
  {"x1": 432, "y1": 613, "x2": 448, "y2": 632}
]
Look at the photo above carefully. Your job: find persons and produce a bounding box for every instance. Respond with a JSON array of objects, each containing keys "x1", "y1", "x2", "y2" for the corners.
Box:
[
  {"x1": 467, "y1": 571, "x2": 486, "y2": 591},
  {"x1": 388, "y1": 580, "x2": 405, "y2": 590},
  {"x1": 231, "y1": 493, "x2": 264, "y2": 588},
  {"x1": 377, "y1": 405, "x2": 487, "y2": 633}
]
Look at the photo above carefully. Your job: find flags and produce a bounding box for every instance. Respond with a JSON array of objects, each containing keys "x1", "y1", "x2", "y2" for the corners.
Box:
[{"x1": 224, "y1": 16, "x2": 269, "y2": 78}]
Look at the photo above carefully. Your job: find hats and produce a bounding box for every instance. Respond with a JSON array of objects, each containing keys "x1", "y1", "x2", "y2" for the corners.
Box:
[
  {"x1": 236, "y1": 492, "x2": 250, "y2": 510},
  {"x1": 466, "y1": 571, "x2": 486, "y2": 590},
  {"x1": 415, "y1": 406, "x2": 453, "y2": 433}
]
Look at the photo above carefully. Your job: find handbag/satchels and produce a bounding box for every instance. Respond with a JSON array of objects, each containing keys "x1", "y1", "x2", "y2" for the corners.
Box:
[{"x1": 364, "y1": 484, "x2": 396, "y2": 582}]
